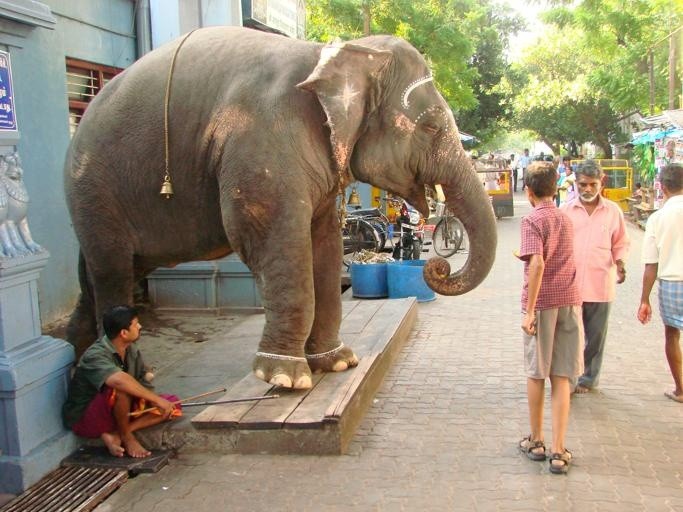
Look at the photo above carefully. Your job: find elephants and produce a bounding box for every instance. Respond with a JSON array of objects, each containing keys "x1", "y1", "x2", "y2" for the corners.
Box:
[{"x1": 63, "y1": 27, "x2": 496, "y2": 391}]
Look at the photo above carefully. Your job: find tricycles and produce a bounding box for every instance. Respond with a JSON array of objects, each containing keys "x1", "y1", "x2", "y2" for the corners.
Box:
[
  {"x1": 570, "y1": 159, "x2": 632, "y2": 213},
  {"x1": 473, "y1": 169, "x2": 514, "y2": 216}
]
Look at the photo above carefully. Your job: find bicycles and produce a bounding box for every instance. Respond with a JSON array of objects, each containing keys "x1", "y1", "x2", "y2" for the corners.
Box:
[
  {"x1": 342, "y1": 205, "x2": 387, "y2": 253},
  {"x1": 431, "y1": 202, "x2": 463, "y2": 258},
  {"x1": 342, "y1": 216, "x2": 379, "y2": 272}
]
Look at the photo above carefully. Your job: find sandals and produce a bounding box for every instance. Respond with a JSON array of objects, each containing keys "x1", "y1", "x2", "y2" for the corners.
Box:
[
  {"x1": 517, "y1": 434, "x2": 546, "y2": 461},
  {"x1": 549, "y1": 449, "x2": 574, "y2": 476}
]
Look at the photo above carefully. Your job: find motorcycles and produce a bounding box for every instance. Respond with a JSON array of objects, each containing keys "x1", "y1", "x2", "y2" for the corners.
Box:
[{"x1": 382, "y1": 198, "x2": 432, "y2": 260}]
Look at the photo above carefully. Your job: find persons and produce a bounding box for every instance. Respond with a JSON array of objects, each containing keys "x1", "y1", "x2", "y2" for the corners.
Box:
[
  {"x1": 538, "y1": 152, "x2": 552, "y2": 161},
  {"x1": 553, "y1": 156, "x2": 576, "y2": 207},
  {"x1": 514, "y1": 162, "x2": 584, "y2": 473},
  {"x1": 521, "y1": 149, "x2": 531, "y2": 192},
  {"x1": 488, "y1": 154, "x2": 511, "y2": 170},
  {"x1": 636, "y1": 163, "x2": 683, "y2": 403},
  {"x1": 557, "y1": 161, "x2": 625, "y2": 395},
  {"x1": 63, "y1": 305, "x2": 183, "y2": 459},
  {"x1": 632, "y1": 183, "x2": 642, "y2": 216},
  {"x1": 506, "y1": 153, "x2": 518, "y2": 192}
]
